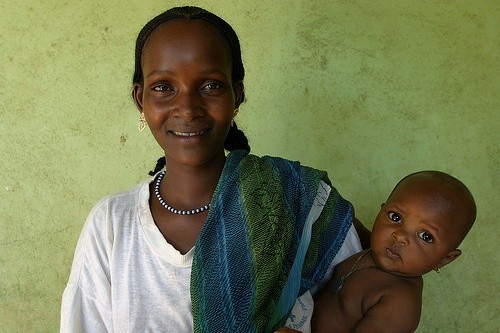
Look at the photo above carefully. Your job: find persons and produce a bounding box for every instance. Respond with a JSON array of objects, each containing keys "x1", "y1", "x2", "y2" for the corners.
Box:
[
  {"x1": 276, "y1": 171, "x2": 479, "y2": 332},
  {"x1": 58, "y1": 4, "x2": 377, "y2": 333}
]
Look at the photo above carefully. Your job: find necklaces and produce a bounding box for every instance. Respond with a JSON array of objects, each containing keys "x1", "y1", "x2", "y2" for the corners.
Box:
[
  {"x1": 153, "y1": 165, "x2": 212, "y2": 214},
  {"x1": 328, "y1": 243, "x2": 381, "y2": 294}
]
